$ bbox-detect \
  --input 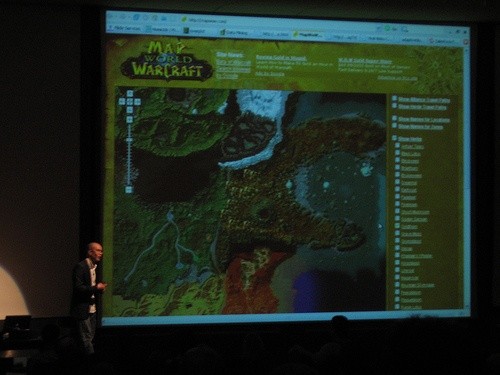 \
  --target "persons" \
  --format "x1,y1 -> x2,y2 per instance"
74,242 -> 108,375
